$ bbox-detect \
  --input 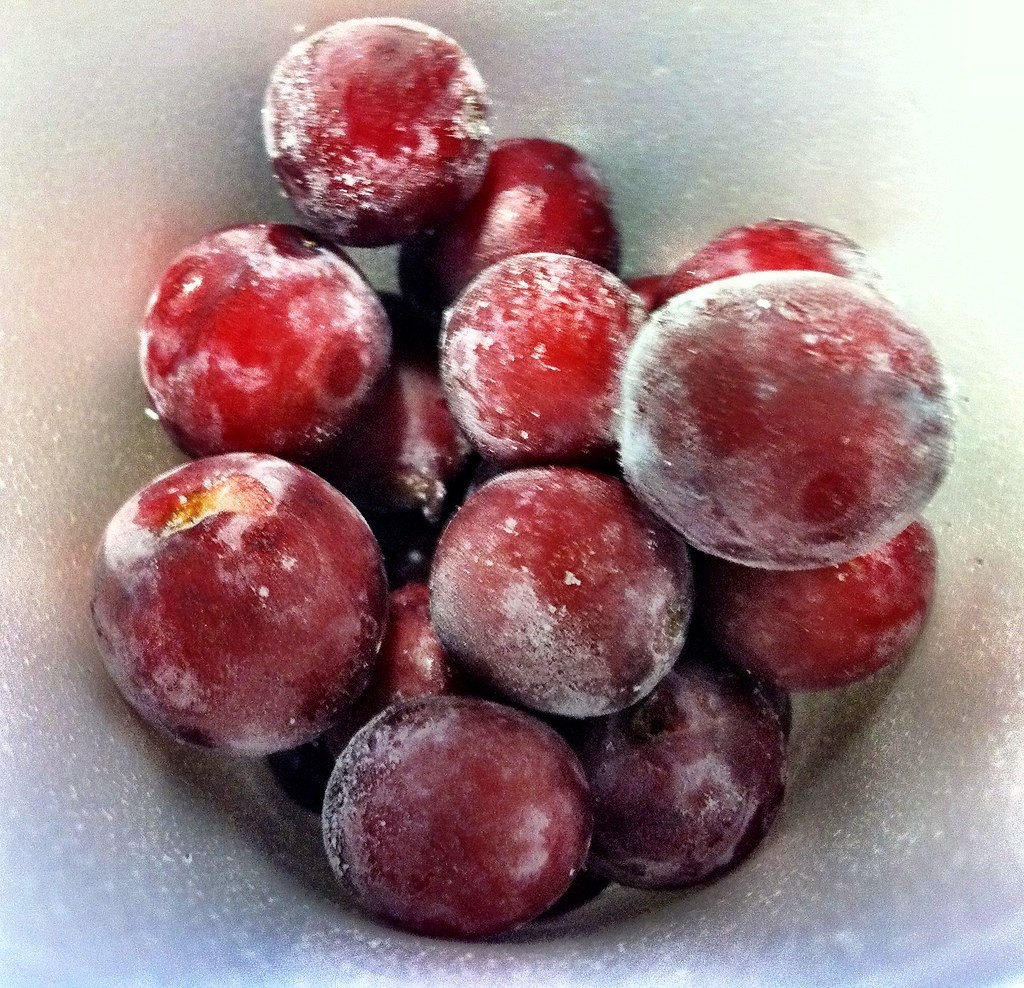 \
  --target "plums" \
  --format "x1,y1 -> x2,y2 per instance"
93,18 -> 954,939
